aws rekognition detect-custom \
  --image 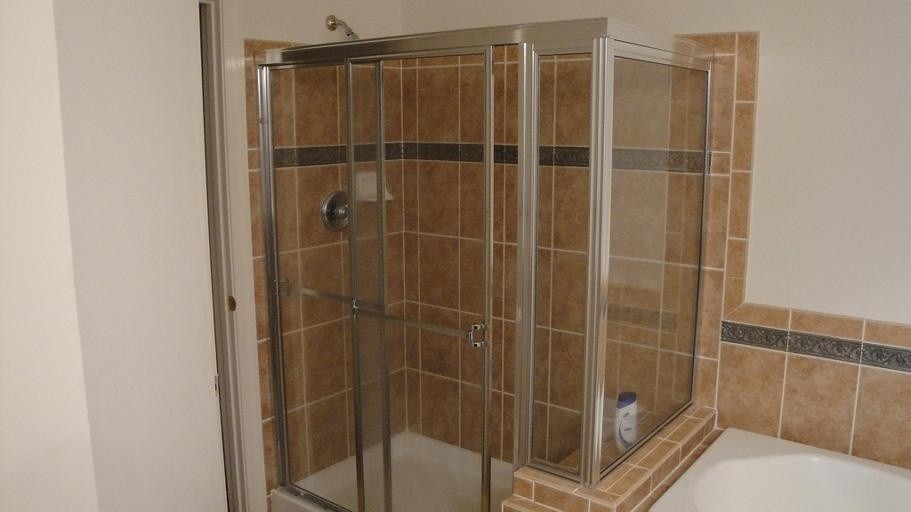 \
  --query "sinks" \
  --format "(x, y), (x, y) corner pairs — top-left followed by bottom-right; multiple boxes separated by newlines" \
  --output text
(648, 428), (910, 512)
